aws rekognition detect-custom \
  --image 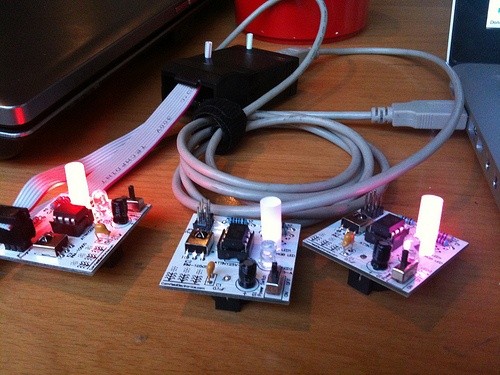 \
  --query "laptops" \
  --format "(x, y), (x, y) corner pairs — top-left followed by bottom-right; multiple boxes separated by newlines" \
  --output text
(449, 0), (500, 214)
(0, 0), (228, 157)
